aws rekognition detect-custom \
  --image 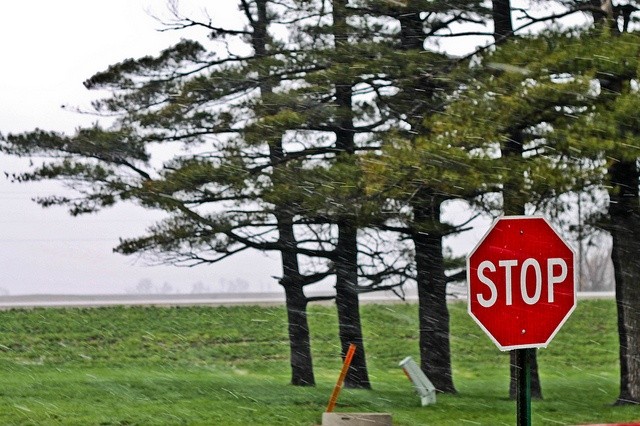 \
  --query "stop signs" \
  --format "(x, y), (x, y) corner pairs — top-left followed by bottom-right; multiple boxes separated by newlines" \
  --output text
(466, 217), (578, 353)
